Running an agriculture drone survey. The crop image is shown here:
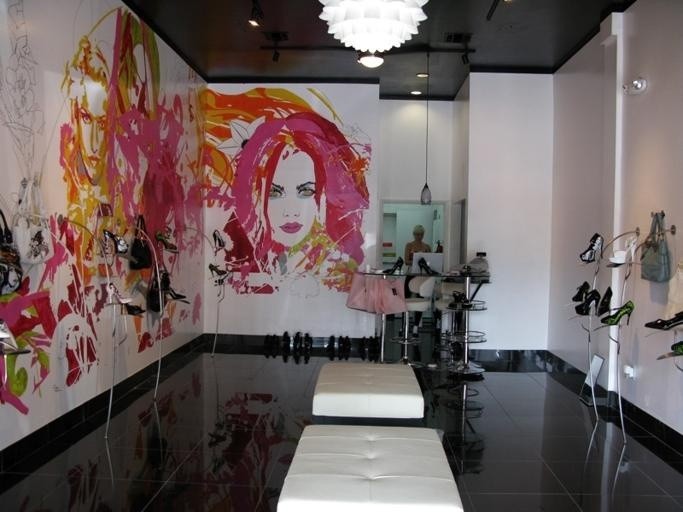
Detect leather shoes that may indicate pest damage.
[597,286,612,317]
[644,310,682,330]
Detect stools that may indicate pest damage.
[279,362,466,512]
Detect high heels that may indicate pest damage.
[575,289,602,316]
[155,231,178,250]
[600,300,635,326]
[108,282,133,304]
[120,301,146,316]
[571,281,590,302]
[418,257,442,276]
[212,229,225,247]
[383,255,404,274]
[580,232,605,262]
[103,229,128,254]
[208,262,226,277]
[164,288,187,300]
[608,237,638,264]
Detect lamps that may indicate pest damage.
[421,59,431,205]
[315,0,428,67]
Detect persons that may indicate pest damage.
[397,223,433,338]
[67,41,113,186]
[236,110,364,279]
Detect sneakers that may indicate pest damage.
[412,328,419,338]
[262,330,381,364]
[448,358,486,374]
[448,290,473,308]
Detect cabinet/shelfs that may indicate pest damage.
[442,278,493,375]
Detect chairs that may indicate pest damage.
[387,276,435,368]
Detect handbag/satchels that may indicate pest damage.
[442,258,491,277]
[12,180,55,265]
[0,209,24,297]
[146,261,171,313]
[129,215,153,270]
[345,272,409,315]
[640,213,672,283]
